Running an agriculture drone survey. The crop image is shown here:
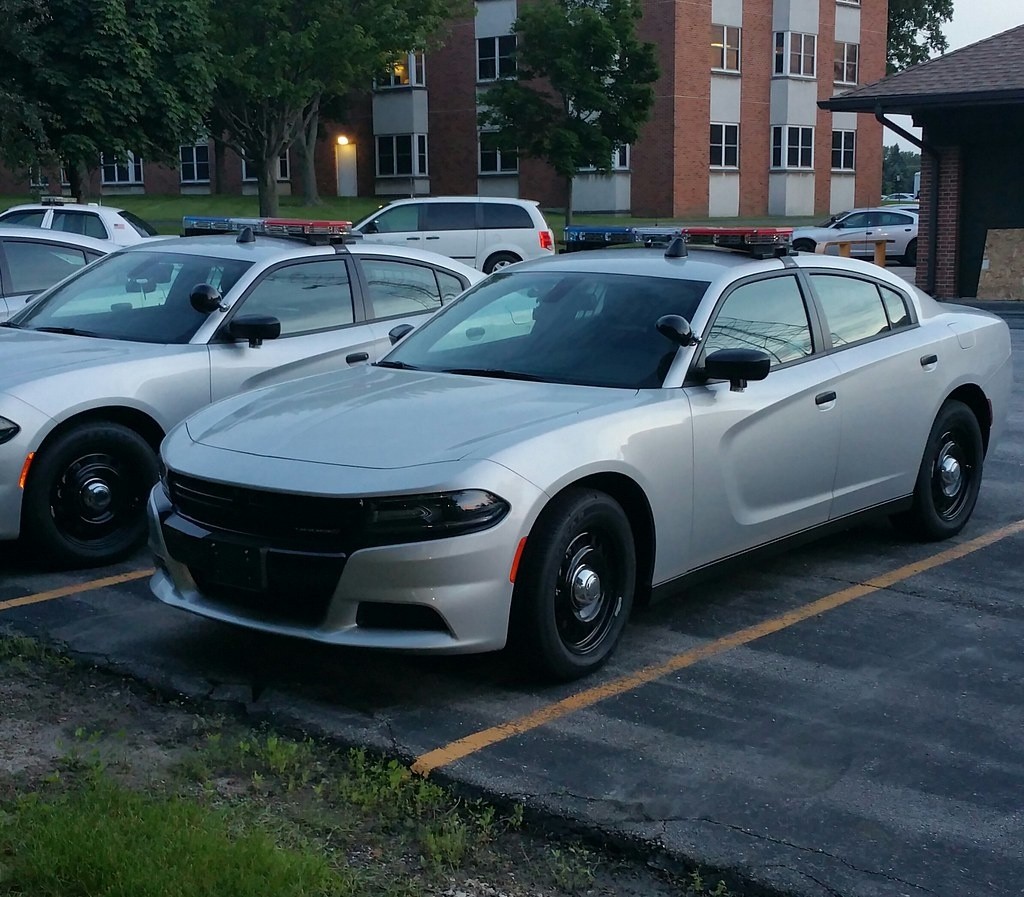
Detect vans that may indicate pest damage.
[356,194,557,275]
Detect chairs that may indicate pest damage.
[184,265,243,337]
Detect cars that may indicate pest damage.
[887,190,910,201]
[147,227,1010,679]
[4,198,155,246]
[2,227,109,314]
[794,205,919,270]
[2,225,495,566]
